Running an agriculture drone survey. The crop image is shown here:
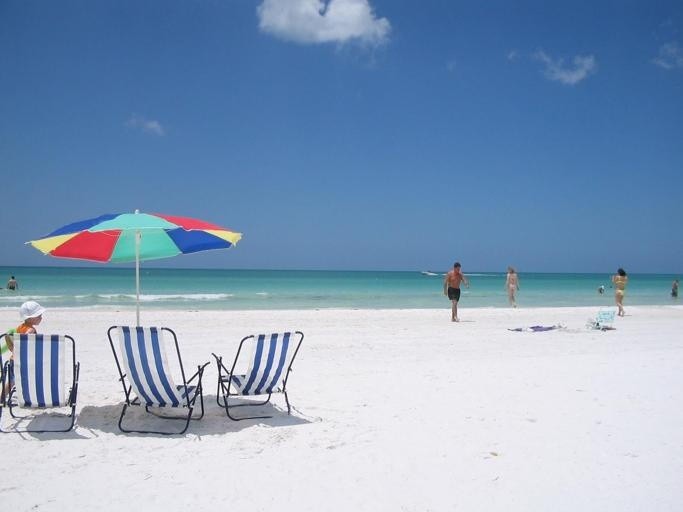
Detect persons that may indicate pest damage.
[6,275,19,291]
[671,279,679,296]
[504,267,520,308]
[443,262,470,322]
[611,267,628,317]
[599,285,605,297]
[0,301,45,400]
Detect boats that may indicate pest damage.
[421,271,433,275]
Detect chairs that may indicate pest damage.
[0,331,79,434]
[108,324,210,435]
[209,330,303,422]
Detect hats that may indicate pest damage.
[20,301,46,322]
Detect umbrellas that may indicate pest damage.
[25,208,243,326]
